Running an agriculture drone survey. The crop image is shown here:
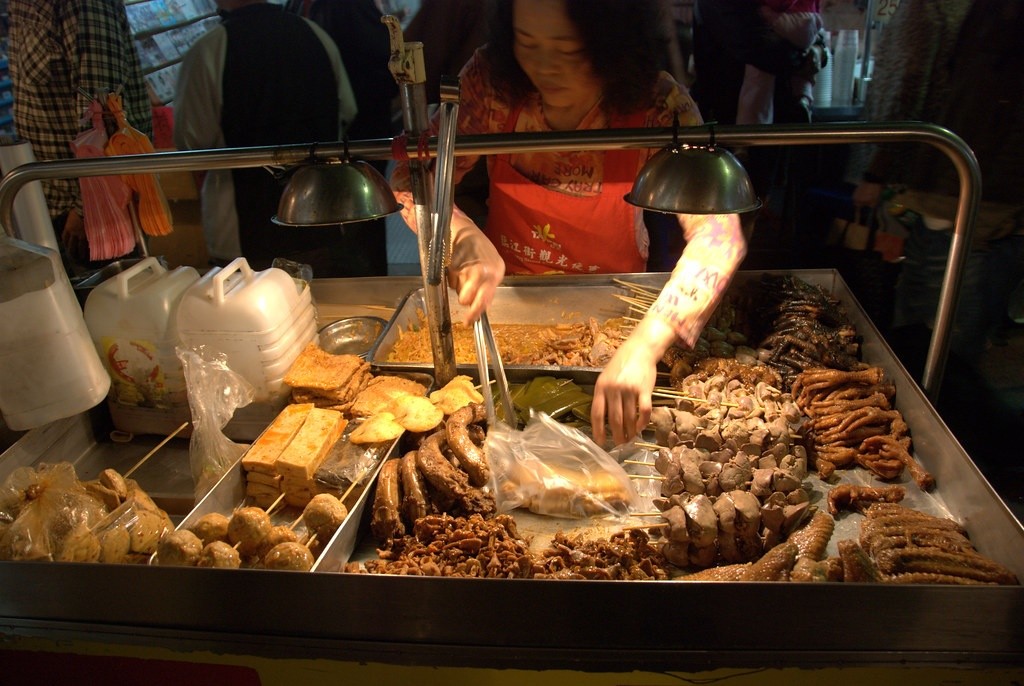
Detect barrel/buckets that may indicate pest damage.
[84,256,319,439]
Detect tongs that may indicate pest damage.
[474,307,518,435]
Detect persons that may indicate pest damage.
[7,0,156,263]
[173,0,358,279]
[387,0,750,449]
[283,0,406,200]
[686,0,830,161]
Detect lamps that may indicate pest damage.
[621,120,766,214]
[271,140,405,229]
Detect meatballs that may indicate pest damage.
[158,494,348,571]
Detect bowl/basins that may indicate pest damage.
[316,314,390,359]
